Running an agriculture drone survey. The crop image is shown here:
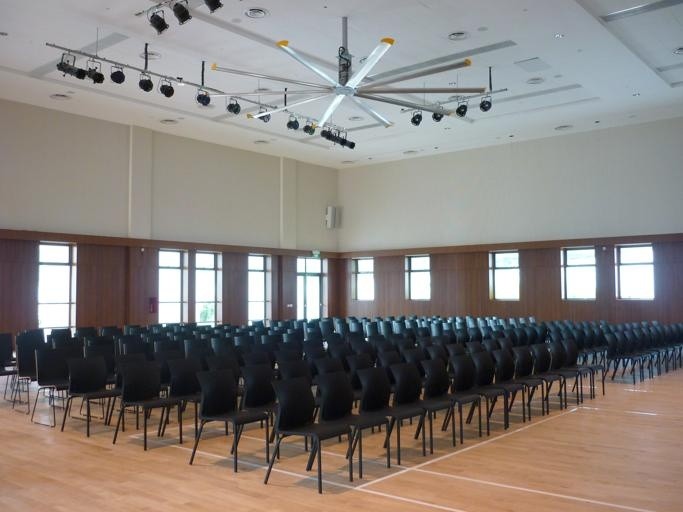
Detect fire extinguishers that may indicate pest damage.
[152,297,158,313]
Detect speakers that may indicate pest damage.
[327,204,338,231]
